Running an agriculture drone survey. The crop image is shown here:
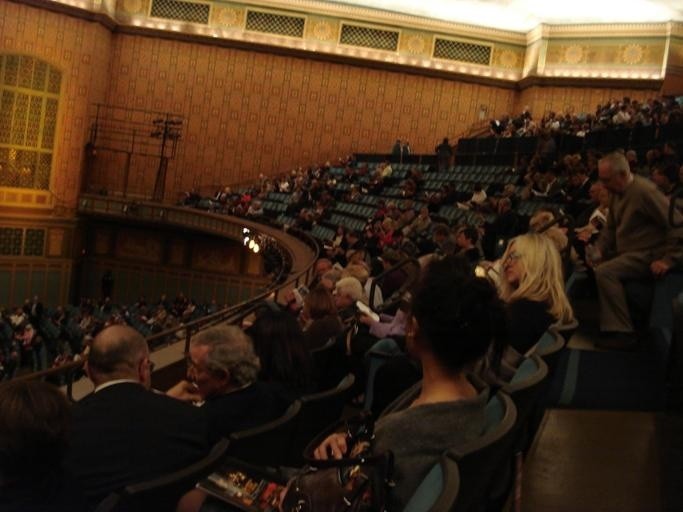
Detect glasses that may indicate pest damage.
[504,254,523,265]
[148,360,155,371]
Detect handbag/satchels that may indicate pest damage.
[281,410,381,512]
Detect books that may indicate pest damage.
[200,462,289,507]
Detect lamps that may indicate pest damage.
[238,227,269,256]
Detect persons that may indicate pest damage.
[298,287,344,352]
[340,263,368,304]
[403,141,412,157]
[332,277,367,327]
[167,326,291,442]
[0,295,41,379]
[41,292,97,372]
[320,271,341,287]
[593,141,682,340]
[41,328,208,512]
[393,139,401,157]
[0,380,70,464]
[315,257,332,276]
[487,91,683,154]
[97,287,195,328]
[322,228,491,261]
[492,234,577,382]
[529,213,577,275]
[435,136,453,159]
[357,290,414,340]
[180,261,530,512]
